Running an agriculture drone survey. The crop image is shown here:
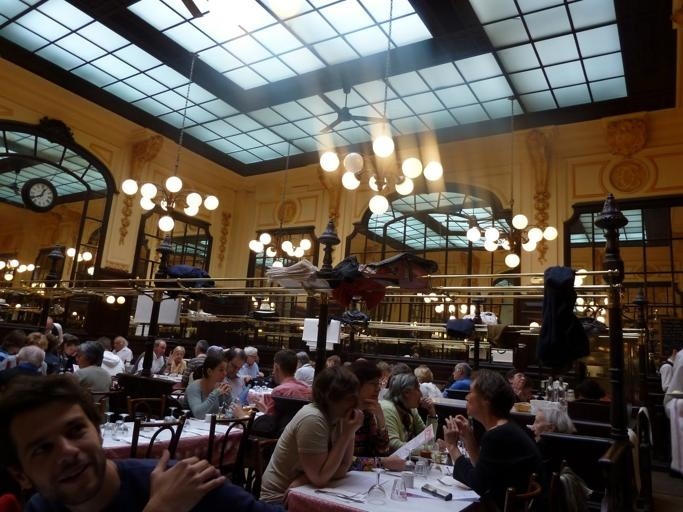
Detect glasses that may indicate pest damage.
[366,381,382,386]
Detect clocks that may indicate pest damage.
[21,177,58,213]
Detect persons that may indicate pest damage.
[231,349,313,465]
[394,363,412,374]
[188,345,224,387]
[378,362,392,388]
[348,360,406,471]
[132,339,177,376]
[258,365,365,512]
[659,343,683,391]
[223,348,248,408]
[0,328,80,375]
[414,365,442,398]
[506,369,533,402]
[0,373,286,512]
[325,355,367,367]
[378,373,438,455]
[294,351,315,386]
[180,340,209,388]
[97,336,126,377]
[442,363,473,397]
[376,365,384,389]
[575,378,605,401]
[72,341,112,410]
[663,349,683,419]
[443,369,541,512]
[531,406,577,443]
[238,346,266,378]
[112,336,133,365]
[183,357,233,418]
[171,346,187,373]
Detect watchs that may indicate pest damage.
[428,414,439,419]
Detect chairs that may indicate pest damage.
[551,459,567,507]
[130,415,186,459]
[207,410,256,483]
[94,398,107,423]
[251,438,279,494]
[504,472,541,512]
[126,394,166,422]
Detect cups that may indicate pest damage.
[182,410,190,432]
[103,412,114,437]
[405,447,415,466]
[120,414,129,435]
[415,461,426,474]
[391,479,407,501]
[368,468,386,503]
[169,407,177,423]
[114,421,124,439]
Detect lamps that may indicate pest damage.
[121,54,219,232]
[466,96,559,269]
[317,0,444,215]
[248,142,311,268]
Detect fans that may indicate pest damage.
[317,84,392,133]
